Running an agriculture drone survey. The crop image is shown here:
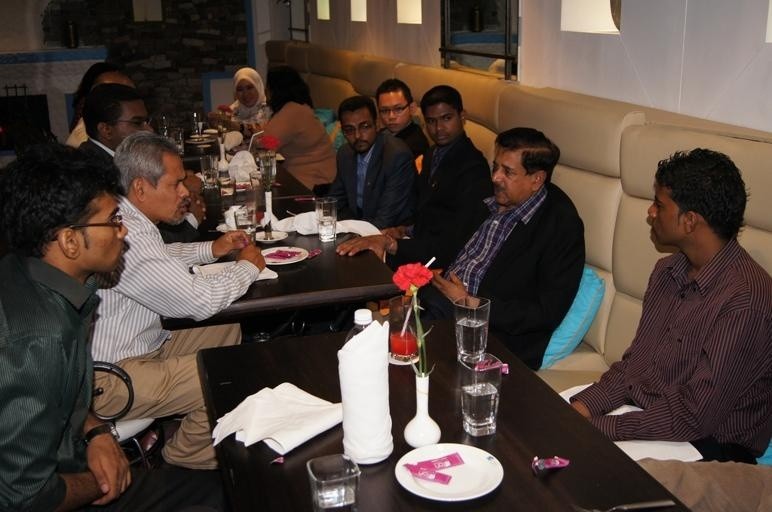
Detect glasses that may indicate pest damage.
[110,117,153,128]
[340,121,377,135]
[379,103,411,115]
[51,207,125,241]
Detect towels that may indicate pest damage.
[274,212,324,234]
[338,318,393,465]
[212,382,343,456]
[336,219,382,237]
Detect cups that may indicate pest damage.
[306,454,361,512]
[316,197,338,242]
[236,210,255,244]
[453,295,490,368]
[459,354,502,436]
[200,155,219,186]
[389,296,420,361]
[170,128,184,157]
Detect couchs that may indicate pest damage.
[263,41,771,393]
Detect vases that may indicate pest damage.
[403,373,441,449]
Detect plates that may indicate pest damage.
[186,138,215,144]
[261,247,309,265]
[395,443,503,501]
[260,150,276,177]
[256,231,287,243]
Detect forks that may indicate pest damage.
[573,499,675,511]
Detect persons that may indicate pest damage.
[569,148,772,465]
[253,65,337,196]
[327,95,417,230]
[0,141,183,512]
[310,78,430,197]
[90,130,265,505]
[226,67,274,143]
[77,82,155,197]
[66,62,137,149]
[332,126,586,372]
[633,458,772,512]
[381,85,494,277]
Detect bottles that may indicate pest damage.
[345,309,375,346]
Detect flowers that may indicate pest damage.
[389,261,439,378]
